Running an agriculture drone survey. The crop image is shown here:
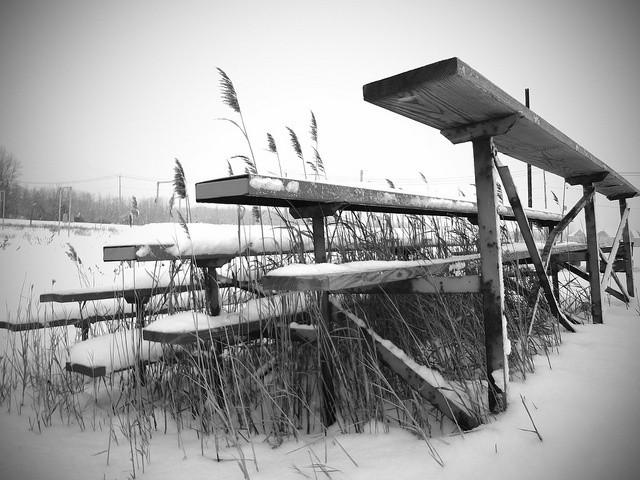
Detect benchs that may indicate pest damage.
[65,229,478,419]
[0,273,278,342]
[145,174,567,427]
[262,57,640,431]
